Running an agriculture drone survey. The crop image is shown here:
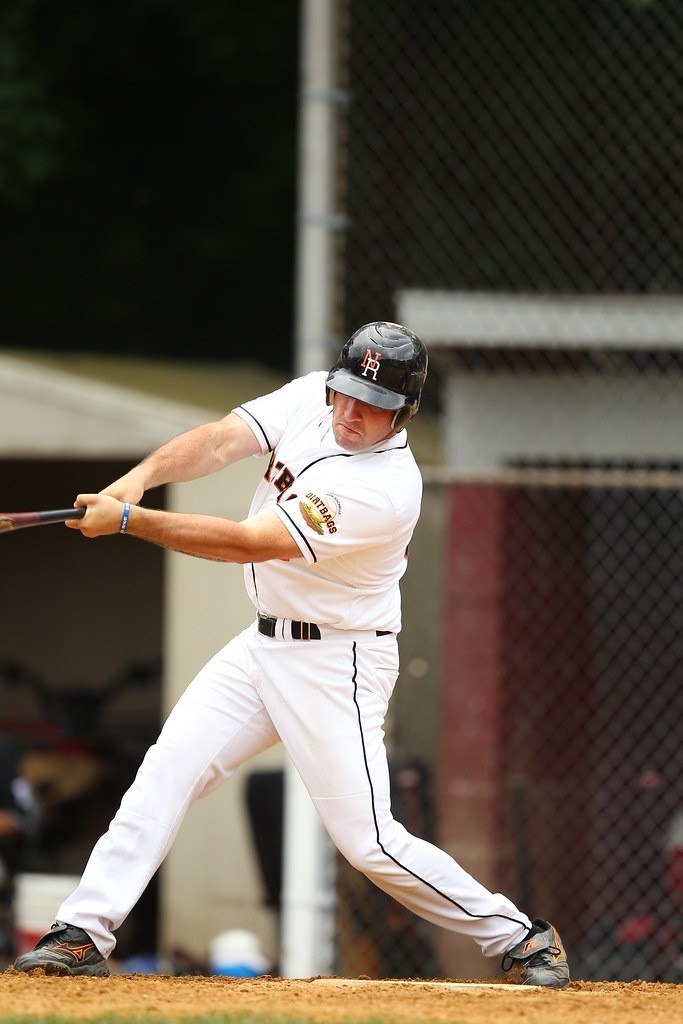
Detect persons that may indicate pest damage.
[14,321,570,989]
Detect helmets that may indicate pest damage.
[326,321,428,432]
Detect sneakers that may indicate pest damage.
[14,922,110,976]
[502,919,570,988]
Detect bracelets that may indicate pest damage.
[120,502,129,534]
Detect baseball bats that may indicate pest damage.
[0,506,86,535]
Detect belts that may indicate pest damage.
[258,615,392,640]
